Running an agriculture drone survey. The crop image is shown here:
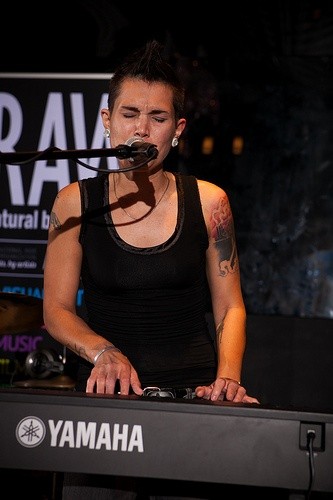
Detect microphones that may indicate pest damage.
[126,136,159,163]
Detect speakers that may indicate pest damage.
[205,312,333,412]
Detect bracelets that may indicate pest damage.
[92,345,121,364]
[218,376,241,384]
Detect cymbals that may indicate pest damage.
[18,375,78,389]
[0,291,45,334]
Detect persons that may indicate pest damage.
[37,38,261,410]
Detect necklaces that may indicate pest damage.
[113,168,170,222]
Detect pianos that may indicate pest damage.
[1,385,333,494]
